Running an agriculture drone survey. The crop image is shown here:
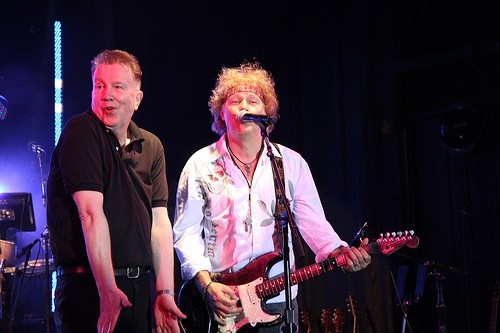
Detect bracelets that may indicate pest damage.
[156,290,175,296]
[203,280,212,300]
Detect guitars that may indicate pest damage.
[0,259,57,275]
[345,293,360,333]
[300,307,312,332]
[320,307,331,333]
[178,231,424,332]
[331,307,344,332]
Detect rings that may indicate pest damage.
[354,262,359,267]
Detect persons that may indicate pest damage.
[172,64,371,333]
[47,49,187,333]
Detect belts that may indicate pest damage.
[56,265,149,279]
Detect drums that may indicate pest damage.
[1,239,17,277]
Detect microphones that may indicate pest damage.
[27,141,46,153]
[350,222,369,248]
[16,239,38,259]
[238,110,274,124]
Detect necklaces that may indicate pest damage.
[227,143,260,231]
